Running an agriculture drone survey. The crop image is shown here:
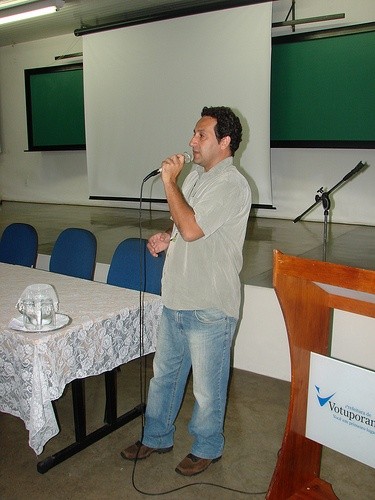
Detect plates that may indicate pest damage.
[7,313,70,333]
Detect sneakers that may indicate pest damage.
[120,440,173,461]
[175,453,222,477]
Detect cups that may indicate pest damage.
[16,283,58,329]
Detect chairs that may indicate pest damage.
[0,222,39,269]
[106,238,166,296]
[48,227,98,282]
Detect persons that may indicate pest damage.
[119,105,252,477]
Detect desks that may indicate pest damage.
[0,261,164,475]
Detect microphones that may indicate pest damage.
[143,152,195,182]
